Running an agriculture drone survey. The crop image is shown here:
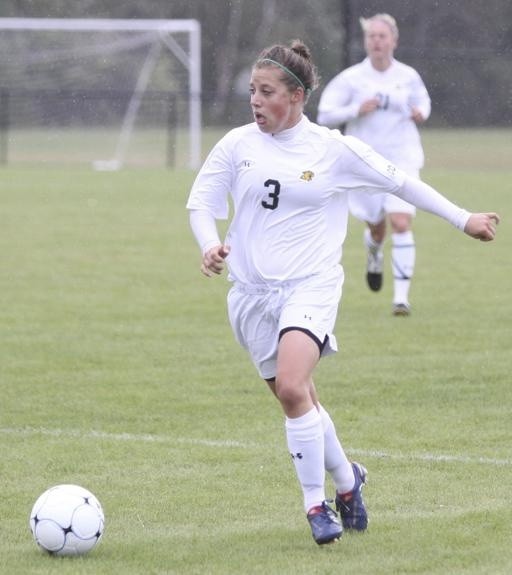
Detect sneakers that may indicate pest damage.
[367,270,383,293]
[307,503,345,547]
[333,460,370,532]
[390,300,411,317]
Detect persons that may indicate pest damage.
[184,38,502,550]
[312,10,435,318]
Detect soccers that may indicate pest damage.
[30,483,105,557]
